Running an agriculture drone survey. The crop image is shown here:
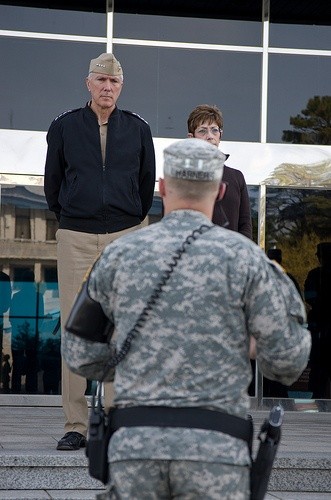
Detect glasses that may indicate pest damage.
[195,128,223,136]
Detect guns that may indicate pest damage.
[251,406,286,500]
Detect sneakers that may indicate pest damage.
[56,432,88,450]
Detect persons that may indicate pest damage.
[161,104,253,241]
[305,242,331,400]
[61,138,311,499]
[44,54,156,451]
[0,265,44,394]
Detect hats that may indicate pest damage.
[314,242,331,254]
[89,52,123,76]
[163,138,225,181]
